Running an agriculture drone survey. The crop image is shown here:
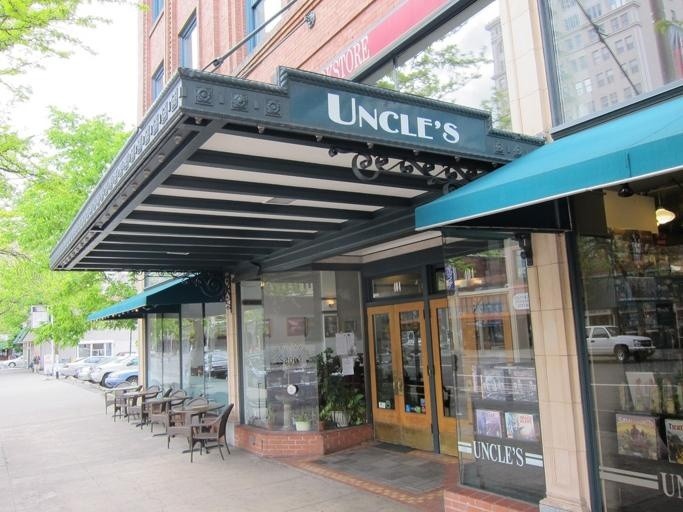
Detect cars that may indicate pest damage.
[7,355,25,368]
[585,324,656,363]
[204,349,228,375]
[61,352,139,387]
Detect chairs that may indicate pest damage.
[104,380,234,463]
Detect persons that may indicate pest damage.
[32,353,40,375]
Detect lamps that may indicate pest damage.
[656,193,676,227]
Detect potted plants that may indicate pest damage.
[294,374,366,431]
